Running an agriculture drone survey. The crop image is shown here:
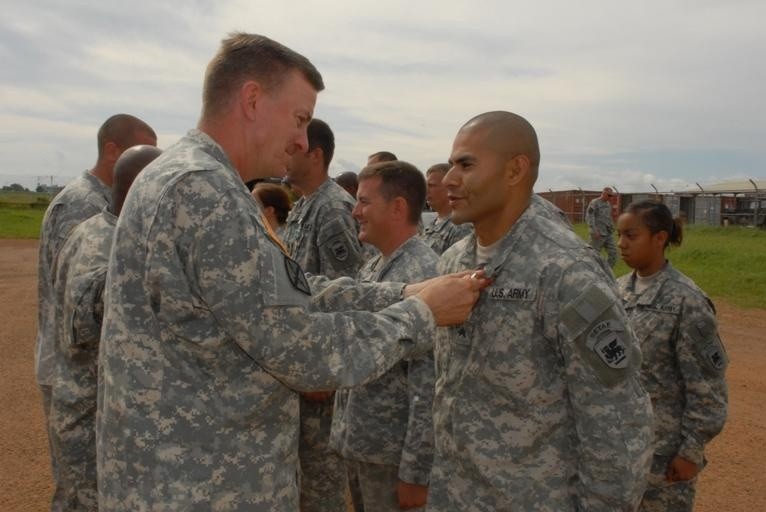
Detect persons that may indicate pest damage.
[48,145,167,511]
[34,115,156,511]
[604,198,729,511]
[96,29,491,511]
[239,118,578,510]
[582,185,618,271]
[430,109,656,512]
